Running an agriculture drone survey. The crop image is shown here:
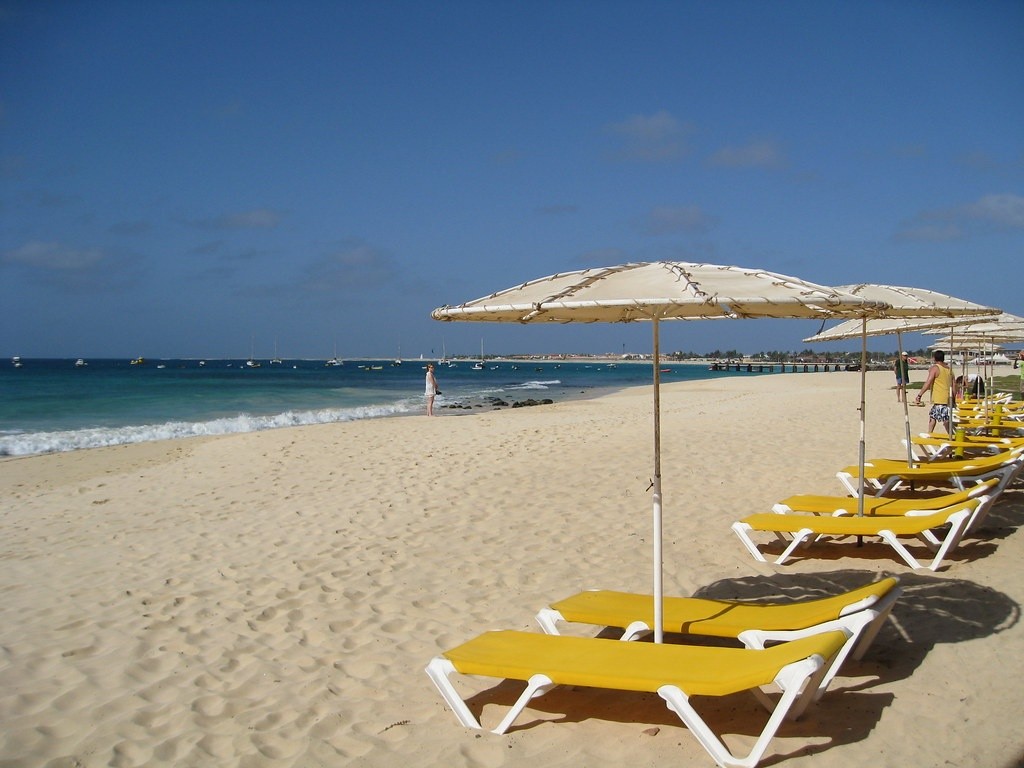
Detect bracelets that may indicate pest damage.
[918,395,921,397]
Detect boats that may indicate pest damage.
[12,356,23,368]
[76,358,88,367]
[131,357,145,365]
[325,344,343,367]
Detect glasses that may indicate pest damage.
[428,366,433,368]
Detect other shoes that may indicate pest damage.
[908,401,925,407]
[436,390,442,395]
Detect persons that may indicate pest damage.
[426,364,438,416]
[1014,351,1024,401]
[894,351,909,402]
[915,350,956,434]
[965,374,984,399]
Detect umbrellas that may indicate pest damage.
[428,261,894,644]
[828,284,1002,517]
[922,312,1024,436]
[802,316,999,491]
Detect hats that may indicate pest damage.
[1020,350,1024,356]
[901,351,908,356]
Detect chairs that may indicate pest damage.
[732,393,1024,571]
[425,626,854,768]
[536,575,903,702]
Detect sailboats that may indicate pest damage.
[472,337,484,369]
[270,335,281,364]
[389,337,402,368]
[439,337,450,366]
[247,335,260,368]
